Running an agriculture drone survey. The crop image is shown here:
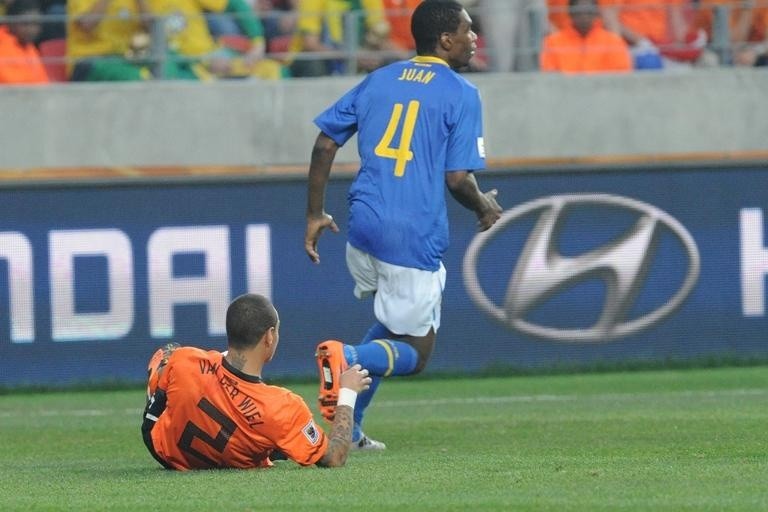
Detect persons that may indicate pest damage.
[142,293,373,472]
[3,1,768,83]
[306,0,503,450]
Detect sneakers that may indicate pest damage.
[316,340,349,424]
[348,434,388,452]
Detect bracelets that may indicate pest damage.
[336,387,358,410]
